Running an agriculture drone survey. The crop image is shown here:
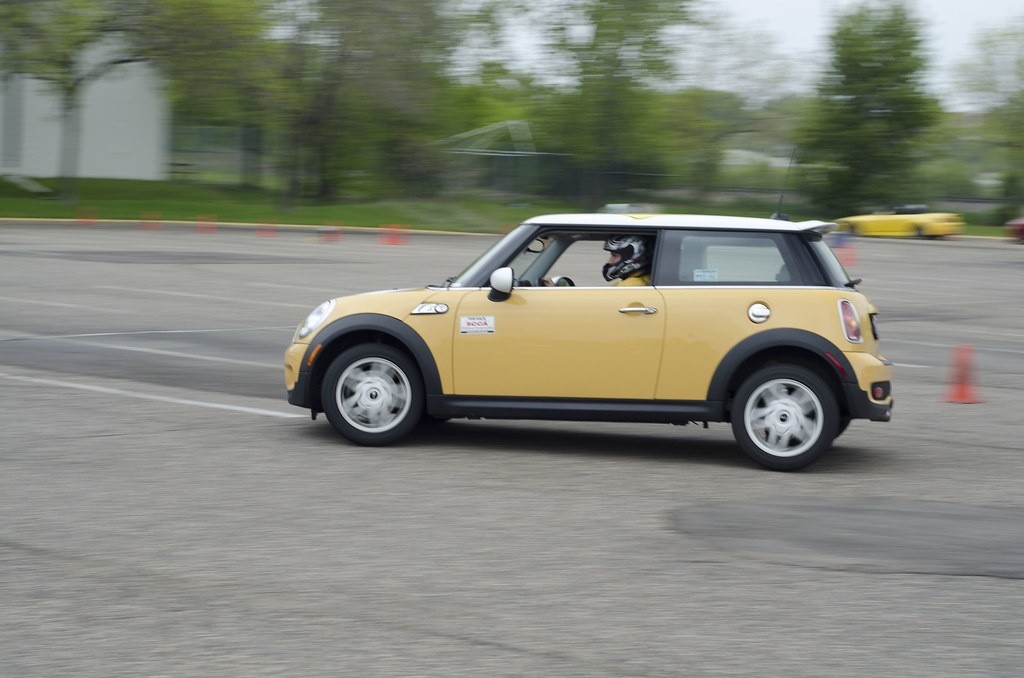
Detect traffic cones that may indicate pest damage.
[943,344,983,404]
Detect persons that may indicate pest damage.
[543,236,651,287]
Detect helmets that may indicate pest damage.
[602,234,651,282]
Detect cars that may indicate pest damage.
[283,211,895,472]
[829,203,969,239]
[1005,215,1024,240]
